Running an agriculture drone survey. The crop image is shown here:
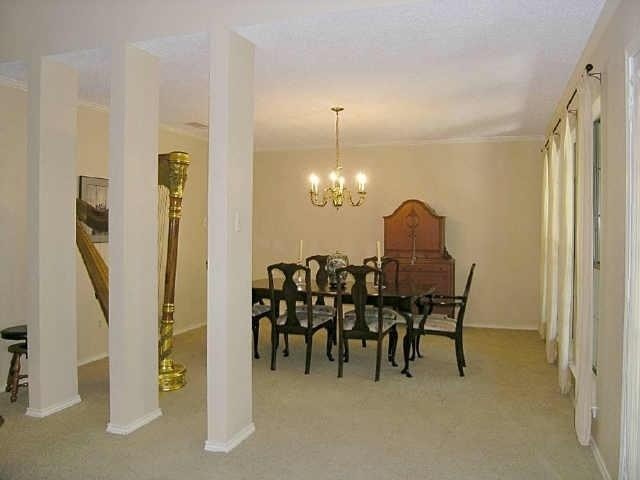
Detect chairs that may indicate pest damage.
[409,263,505,377]
[252,305,279,358]
[335,264,406,382]
[266,262,335,375]
[305,255,337,345]
[361,256,399,347]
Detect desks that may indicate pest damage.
[0,325,27,340]
[252,278,438,377]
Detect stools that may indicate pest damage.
[6,343,27,403]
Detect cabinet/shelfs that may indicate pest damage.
[382,258,455,319]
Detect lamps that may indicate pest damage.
[308,106,367,210]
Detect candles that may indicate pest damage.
[376,240,381,261]
[299,240,303,259]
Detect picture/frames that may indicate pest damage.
[79,176,109,244]
